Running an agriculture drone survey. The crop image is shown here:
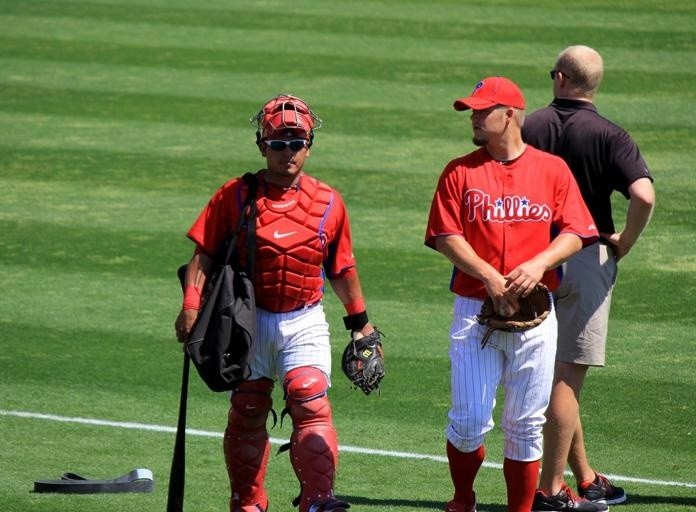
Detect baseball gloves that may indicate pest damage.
[341,327,384,396]
[477,281,551,349]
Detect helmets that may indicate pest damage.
[248,92,323,140]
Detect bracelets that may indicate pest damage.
[181,283,202,312]
[344,295,366,315]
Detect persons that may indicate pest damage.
[175,93,385,512]
[423,76,614,510]
[511,42,657,510]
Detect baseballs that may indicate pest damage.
[499,303,517,316]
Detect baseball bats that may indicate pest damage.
[167,345,189,511]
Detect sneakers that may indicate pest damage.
[532,482,609,512]
[577,471,626,504]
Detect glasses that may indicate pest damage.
[264,138,308,151]
[550,70,570,80]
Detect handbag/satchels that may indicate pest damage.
[178,255,257,392]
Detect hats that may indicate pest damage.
[454,76,526,112]
